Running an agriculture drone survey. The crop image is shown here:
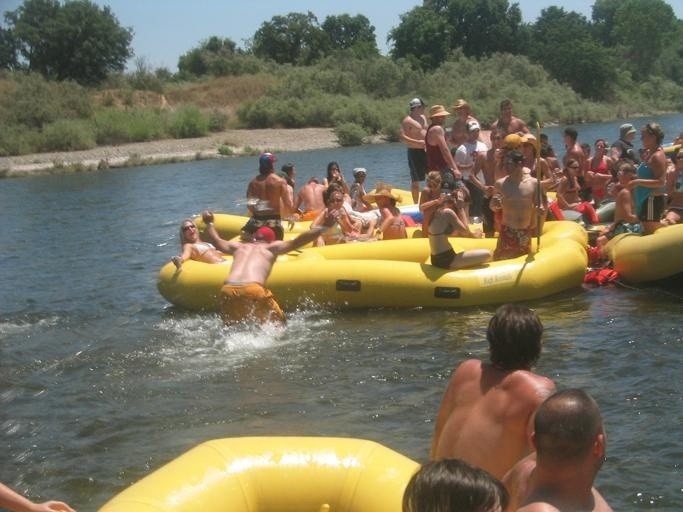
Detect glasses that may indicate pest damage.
[183,225,194,232]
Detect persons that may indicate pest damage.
[203,207,341,329]
[501,388,614,512]
[429,304,557,481]
[172,97,682,269]
[0,483,76,512]
[402,458,511,512]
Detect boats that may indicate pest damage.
[156,235,588,313]
[95,436,423,511]
[366,186,559,210]
[192,213,587,250]
[334,204,585,228]
[590,222,683,285]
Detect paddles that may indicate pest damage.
[535,121,541,249]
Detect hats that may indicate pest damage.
[562,127,577,139]
[500,134,536,151]
[259,152,277,162]
[255,226,275,240]
[362,182,402,203]
[409,97,481,132]
[619,123,636,138]
[352,167,367,175]
